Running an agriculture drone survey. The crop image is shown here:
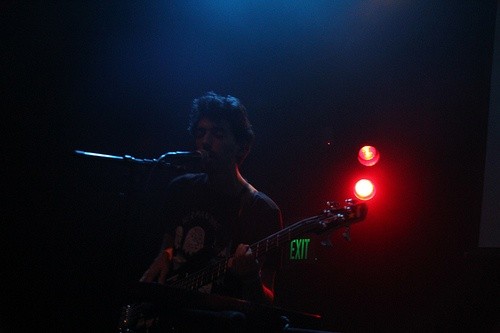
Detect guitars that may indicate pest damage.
[120,199,366,333]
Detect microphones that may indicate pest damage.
[160,149,210,163]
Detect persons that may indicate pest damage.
[126,93,284,332]
